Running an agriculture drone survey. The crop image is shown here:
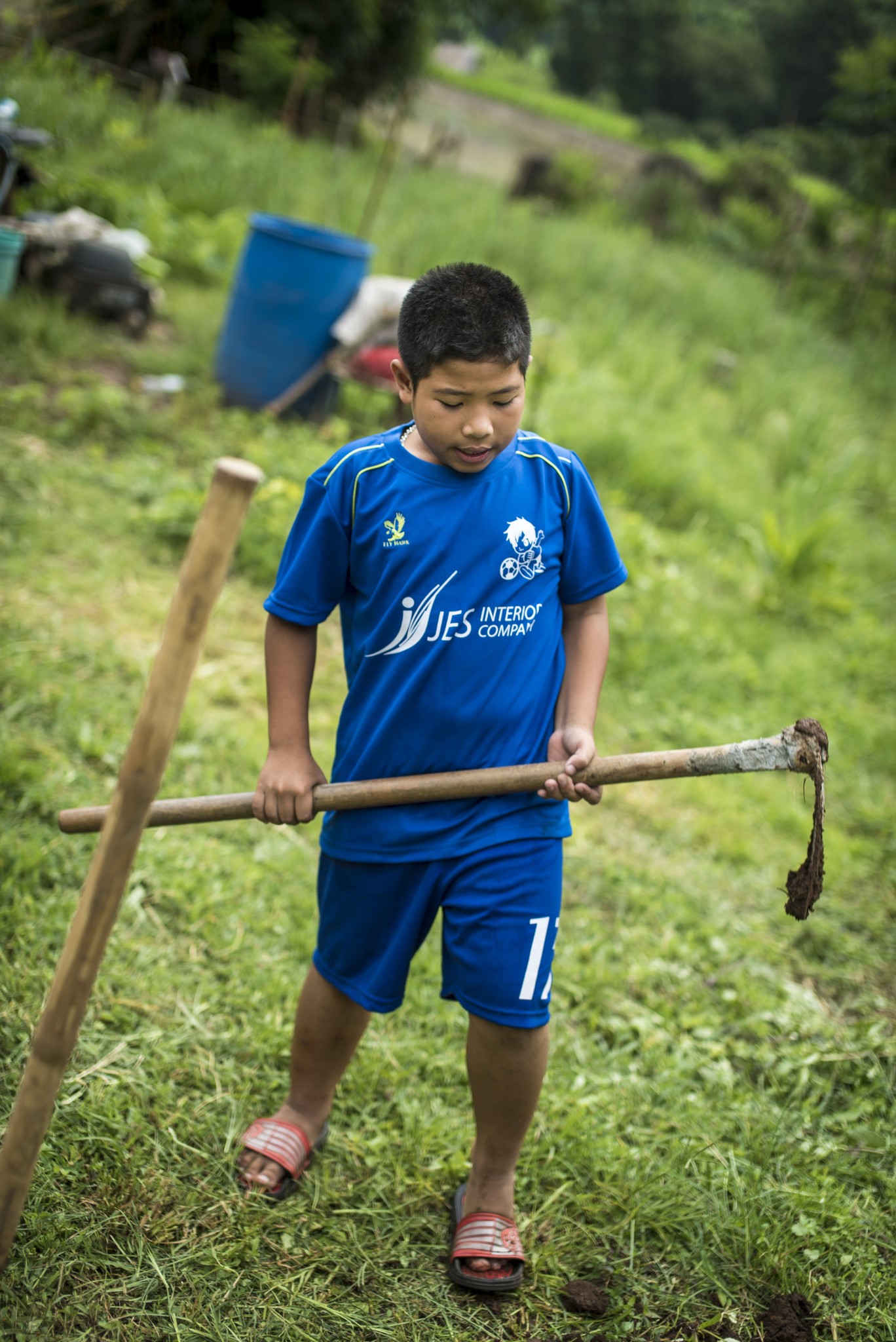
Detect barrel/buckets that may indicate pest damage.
[214,209,375,428]
[1,230,27,299]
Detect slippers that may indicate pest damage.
[235,1115,330,1199]
[449,1181,527,1293]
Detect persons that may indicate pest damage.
[236,261,630,1294]
